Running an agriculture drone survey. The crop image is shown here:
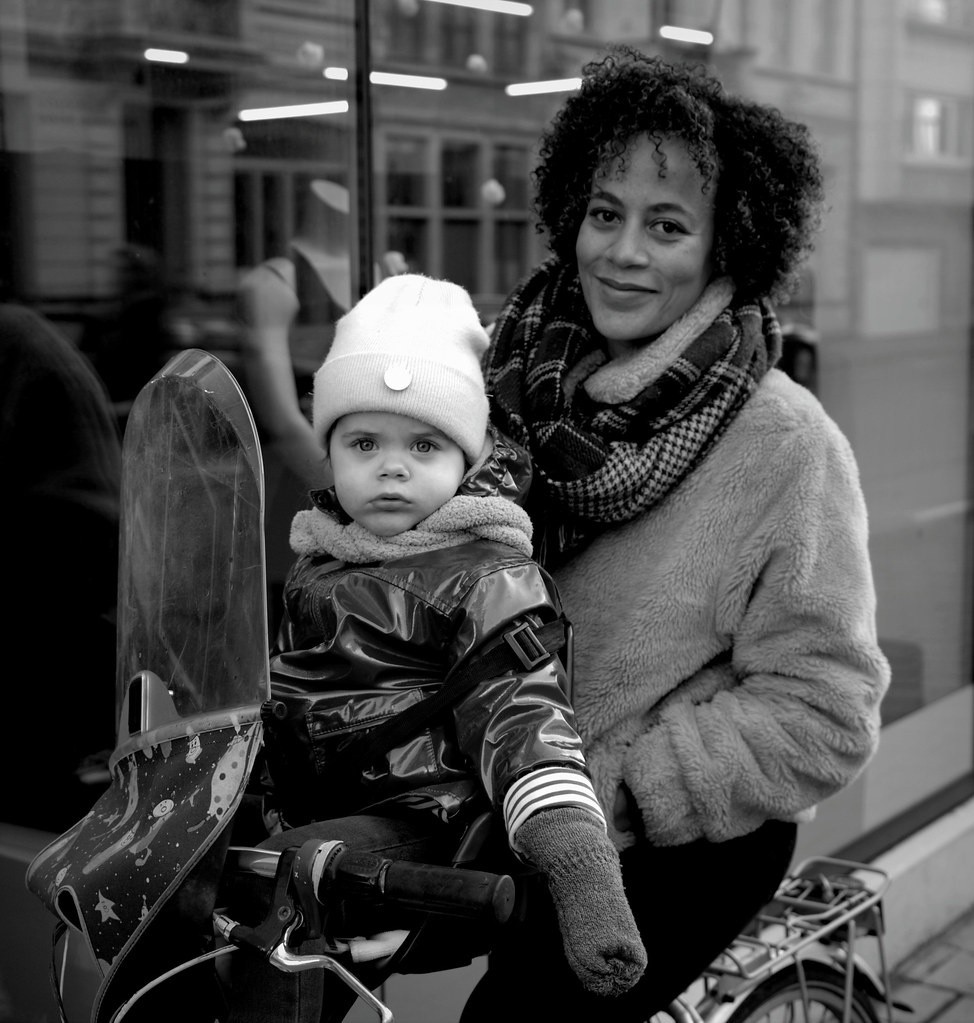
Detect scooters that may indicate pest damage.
[0,347,915,1023]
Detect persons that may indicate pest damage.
[185,45,891,1023]
[236,179,400,676]
[115,265,646,1023]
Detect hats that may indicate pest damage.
[312,273,491,465]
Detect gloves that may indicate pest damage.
[513,808,647,996]
